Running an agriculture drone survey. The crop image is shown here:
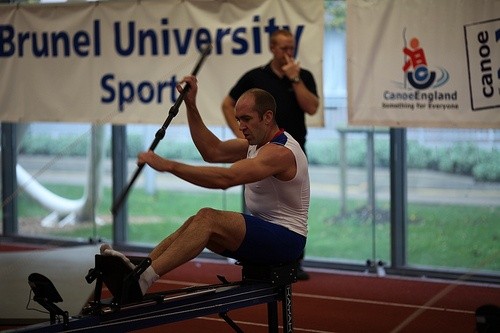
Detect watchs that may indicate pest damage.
[290,77,302,83]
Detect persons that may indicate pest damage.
[221,29,319,281]
[100,88,310,297]
